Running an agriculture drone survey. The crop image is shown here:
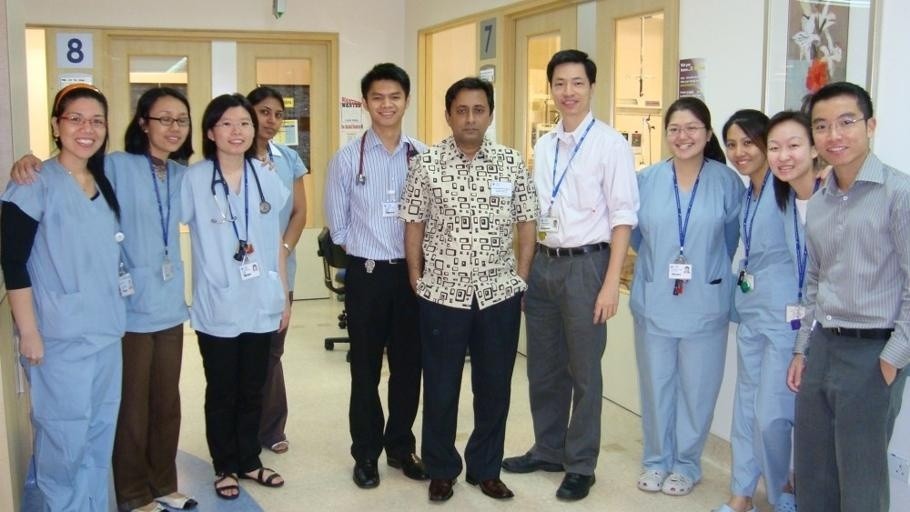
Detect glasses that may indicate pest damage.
[61,117,104,126]
[667,127,705,136]
[813,118,865,133]
[148,117,190,126]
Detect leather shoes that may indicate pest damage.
[353,450,595,501]
[130,496,198,512]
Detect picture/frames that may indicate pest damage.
[761,0,883,150]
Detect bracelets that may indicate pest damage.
[283,241,292,252]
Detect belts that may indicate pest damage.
[832,327,890,338]
[350,257,405,264]
[540,242,608,256]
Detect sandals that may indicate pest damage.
[238,468,284,486]
[215,472,240,499]
[269,439,288,453]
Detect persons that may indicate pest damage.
[400,77,540,503]
[253,264,257,271]
[715,105,801,512]
[552,220,557,227]
[626,93,747,497]
[177,91,293,499]
[328,62,435,492]
[0,82,132,512]
[683,266,689,274]
[780,80,910,512]
[765,107,833,315]
[500,49,643,505]
[245,85,313,458]
[10,87,199,512]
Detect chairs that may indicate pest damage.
[317,226,355,362]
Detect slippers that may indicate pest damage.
[712,503,757,512]
[775,492,797,512]
[638,470,693,495]
